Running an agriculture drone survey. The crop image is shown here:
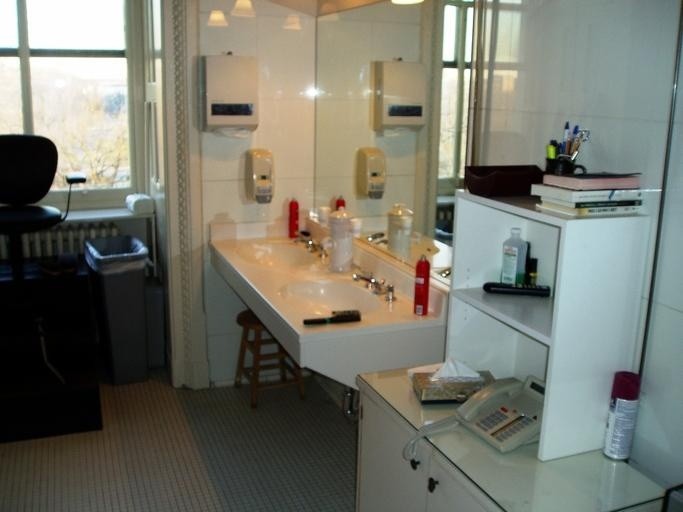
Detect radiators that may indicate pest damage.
[21,223,118,257]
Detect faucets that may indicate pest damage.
[352,273,387,295]
[440,268,452,278]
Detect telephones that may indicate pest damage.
[454,375,545,454]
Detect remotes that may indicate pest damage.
[483,283,550,297]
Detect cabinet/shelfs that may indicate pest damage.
[443,190,652,461]
[354,393,498,512]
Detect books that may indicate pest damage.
[529,170,642,215]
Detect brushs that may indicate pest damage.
[301,310,361,325]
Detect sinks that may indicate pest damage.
[278,280,382,314]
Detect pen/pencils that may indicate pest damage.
[546,118,589,162]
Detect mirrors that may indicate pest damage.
[316,0,477,284]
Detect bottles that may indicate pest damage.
[500,227,539,288]
[327,206,354,275]
[601,371,640,459]
[413,255,431,316]
[287,199,299,239]
[335,196,345,209]
[386,203,413,262]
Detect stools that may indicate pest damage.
[235,309,308,408]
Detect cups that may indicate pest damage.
[544,158,588,177]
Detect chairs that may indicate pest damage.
[1,135,61,277]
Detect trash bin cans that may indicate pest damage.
[84,234,149,388]
